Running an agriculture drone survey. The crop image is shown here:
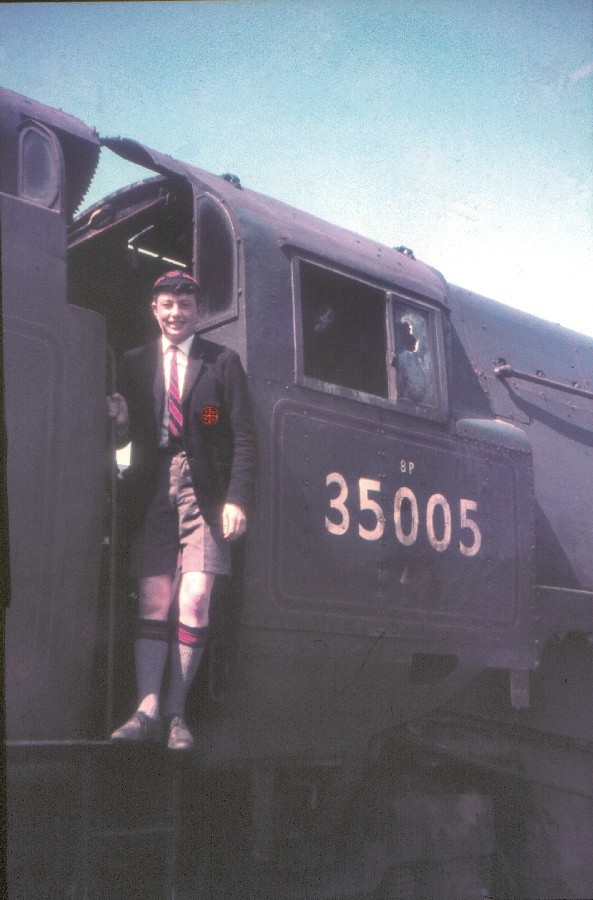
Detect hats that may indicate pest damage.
[153,270,201,293]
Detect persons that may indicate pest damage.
[105,270,259,752]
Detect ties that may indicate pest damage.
[168,346,183,441]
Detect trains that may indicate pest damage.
[0,84,593,900]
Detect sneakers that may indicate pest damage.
[165,712,195,751]
[111,711,163,745]
